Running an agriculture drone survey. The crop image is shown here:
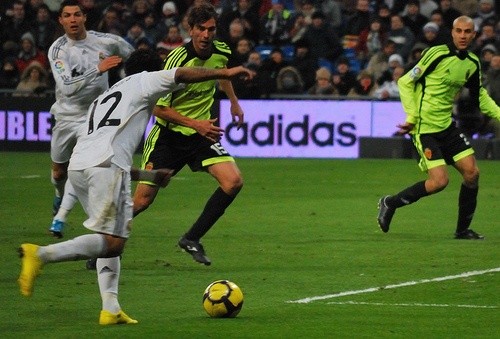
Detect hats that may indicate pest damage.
[423,22,439,34]
[388,54,403,67]
[316,66,330,80]
[162,2,176,14]
[311,12,327,20]
[20,32,35,47]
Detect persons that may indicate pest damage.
[376,15,500,240]
[47,0,135,238]
[85,5,244,266]
[15,48,256,325]
[0,0,500,106]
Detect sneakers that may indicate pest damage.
[453,229,484,241]
[377,195,395,233]
[175,237,212,267]
[98,309,139,326]
[47,219,65,240]
[85,258,96,271]
[51,196,62,215]
[15,241,46,299]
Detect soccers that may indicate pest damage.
[203,279,243,318]
